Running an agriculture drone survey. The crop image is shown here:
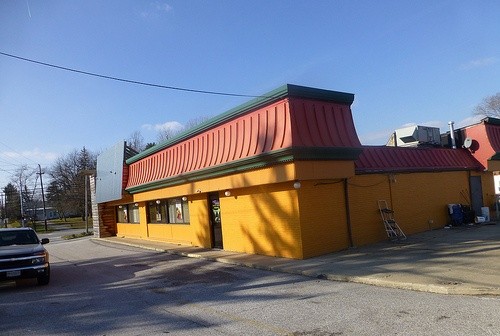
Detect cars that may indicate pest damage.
[0,227,51,285]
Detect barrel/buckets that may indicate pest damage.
[481,207,490,222]
[474,216,486,223]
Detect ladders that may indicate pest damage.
[378,200,407,241]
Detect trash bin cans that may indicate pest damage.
[460,204,472,224]
[448,203,464,226]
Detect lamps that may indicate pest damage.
[293,181,301,189]
[182,196,187,201]
[225,190,230,196]
